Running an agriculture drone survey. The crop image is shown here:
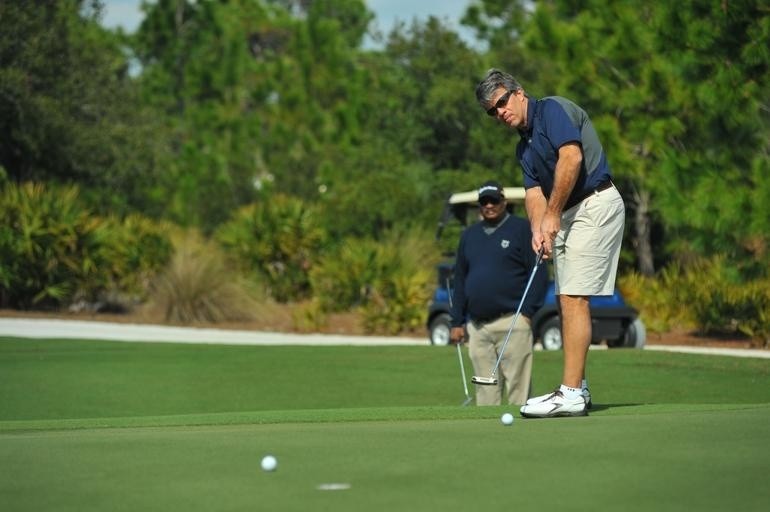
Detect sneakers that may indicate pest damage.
[520,387,591,418]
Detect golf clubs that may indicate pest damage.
[445,278,472,406]
[471,245,544,385]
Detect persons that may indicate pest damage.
[449,180,549,408]
[476,67,628,419]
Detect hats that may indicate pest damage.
[479,182,505,202]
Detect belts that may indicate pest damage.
[557,180,613,212]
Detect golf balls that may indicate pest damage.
[501,413,512,425]
[261,456,276,471]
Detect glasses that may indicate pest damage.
[486,92,513,119]
